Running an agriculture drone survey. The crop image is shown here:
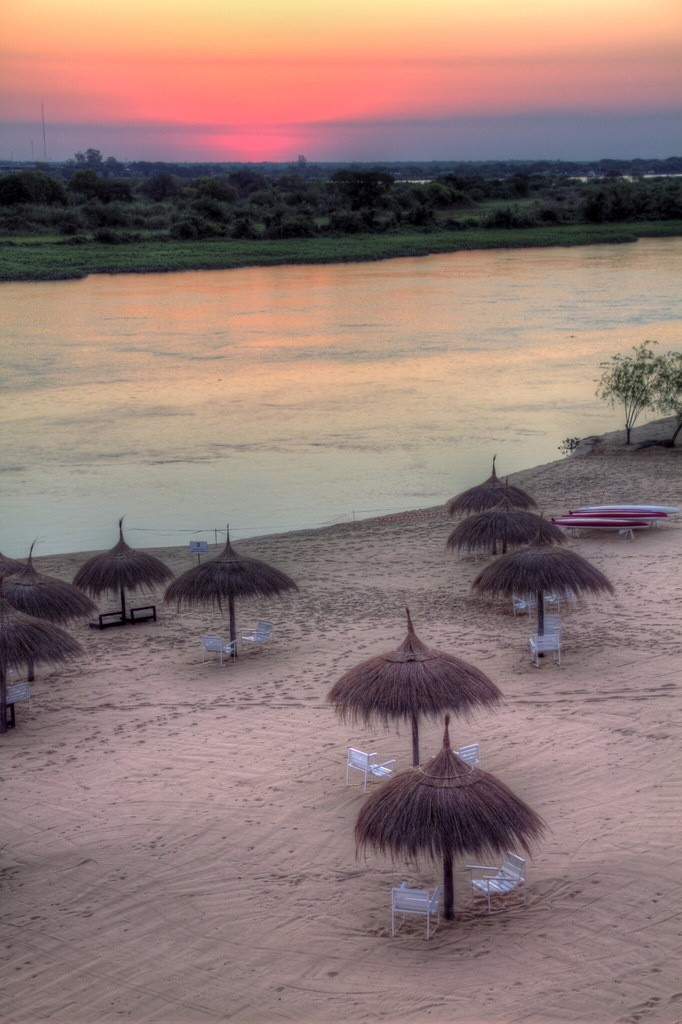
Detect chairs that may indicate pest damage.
[391,881,440,940]
[543,589,561,614]
[89,611,124,630]
[529,634,561,667]
[240,618,275,654]
[347,747,396,793]
[512,589,531,619]
[202,636,237,667]
[544,613,562,636]
[432,743,480,768]
[120,605,157,625]
[5,683,31,722]
[465,851,528,915]
[460,541,485,561]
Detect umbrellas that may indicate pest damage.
[71,517,175,626]
[448,454,539,517]
[444,476,568,554]
[325,606,504,767]
[353,714,551,921]
[164,522,299,658]
[471,511,614,658]
[0,542,101,682]
[0,592,84,732]
[0,551,26,580]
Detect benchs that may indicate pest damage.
[547,504,681,541]
